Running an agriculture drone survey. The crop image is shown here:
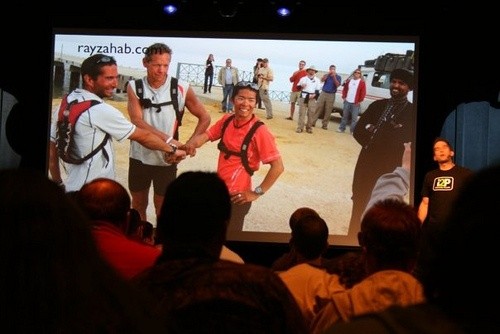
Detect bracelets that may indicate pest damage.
[166,137,173,144]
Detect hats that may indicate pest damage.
[305,65,318,74]
[389,68,414,91]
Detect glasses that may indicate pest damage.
[235,81,260,91]
[96,56,117,65]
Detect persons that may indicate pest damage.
[417,137,472,231]
[0,160,500,334]
[46,43,414,236]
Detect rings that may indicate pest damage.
[238,194,241,198]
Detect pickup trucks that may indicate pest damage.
[330,65,417,121]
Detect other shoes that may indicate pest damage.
[218,110,226,113]
[336,129,345,133]
[295,128,302,133]
[306,128,312,133]
[322,127,328,130]
[228,110,232,114]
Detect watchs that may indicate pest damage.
[169,143,178,154]
[254,186,264,195]
